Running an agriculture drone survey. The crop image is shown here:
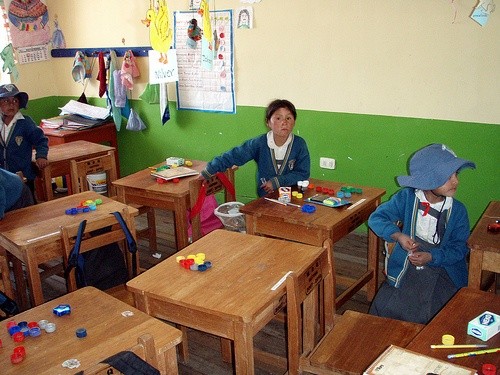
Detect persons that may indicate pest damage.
[0,83,49,189]
[0,168,34,221]
[368,144,477,326]
[194,99,311,197]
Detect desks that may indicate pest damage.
[126,229,322,375]
[238,178,386,337]
[404,285,500,375]
[0,286,183,375]
[32,139,116,202]
[466,200,500,293]
[111,160,239,251]
[38,123,121,197]
[0,191,140,311]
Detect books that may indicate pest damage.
[363,344,479,375]
[151,166,199,181]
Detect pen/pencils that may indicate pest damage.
[447,348,500,359]
[430,344,487,348]
[146,167,156,170]
[264,197,286,205]
[287,202,301,208]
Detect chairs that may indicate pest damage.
[60,207,189,365]
[188,165,236,243]
[286,238,426,375]
[70,150,157,254]
[82,332,161,375]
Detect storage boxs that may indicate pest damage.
[467,311,500,341]
[279,186,291,202]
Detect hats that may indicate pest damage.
[0,84,28,109]
[397,143,476,190]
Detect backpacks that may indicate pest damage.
[187,173,235,244]
[65,212,137,291]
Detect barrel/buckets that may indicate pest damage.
[86,172,108,193]
